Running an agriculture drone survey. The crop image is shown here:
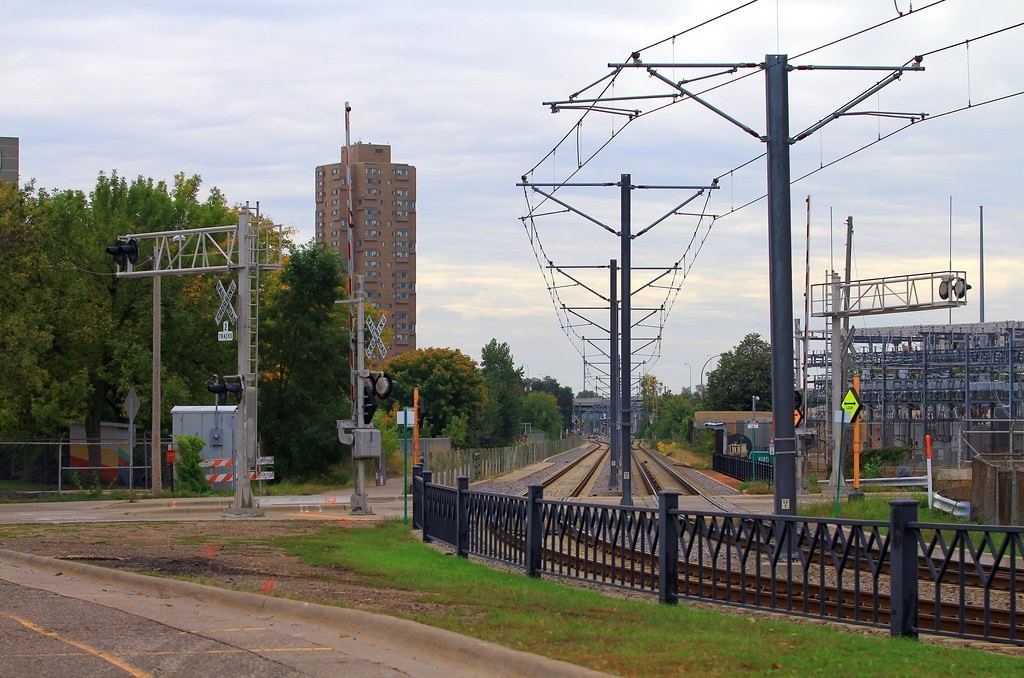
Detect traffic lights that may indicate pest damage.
[105,240,126,266]
[120,239,138,264]
[207,377,227,400]
[225,377,242,403]
